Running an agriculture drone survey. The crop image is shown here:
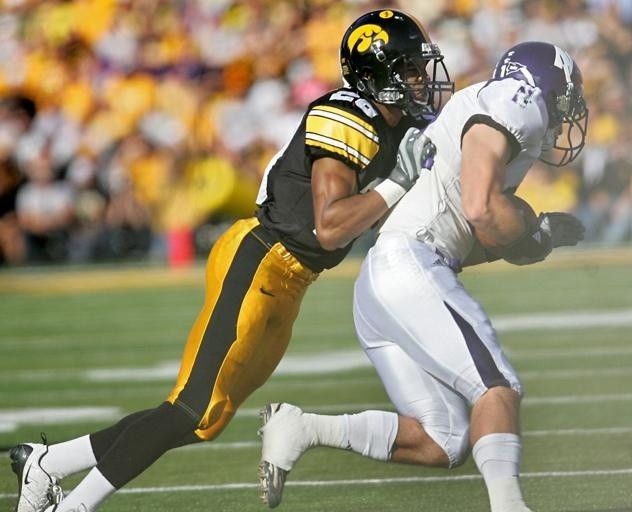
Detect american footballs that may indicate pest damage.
[472,191,542,261]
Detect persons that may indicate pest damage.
[10,7,456,512]
[1,1,632,275]
[256,38,592,512]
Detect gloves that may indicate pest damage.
[498,227,552,266]
[386,126,438,191]
[538,210,585,250]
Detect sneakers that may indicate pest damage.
[257,401,303,509]
[9,432,62,512]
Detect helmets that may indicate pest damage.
[339,9,456,130]
[492,42,589,169]
